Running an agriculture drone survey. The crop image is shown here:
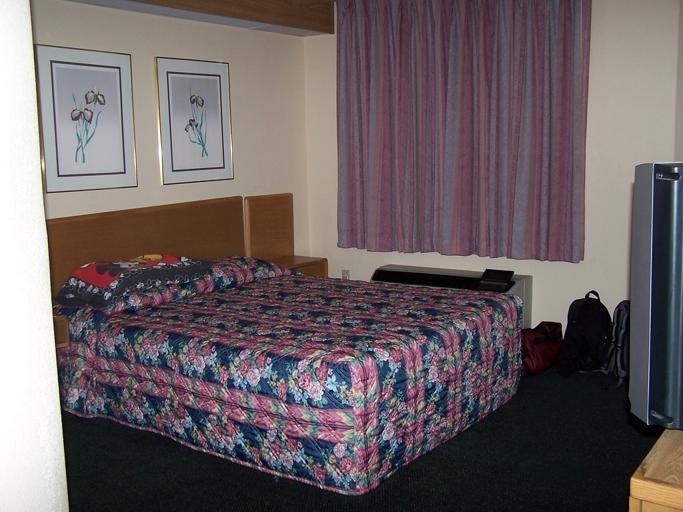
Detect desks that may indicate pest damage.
[628,429,683,511]
[370,264,532,333]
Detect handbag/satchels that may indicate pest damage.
[516,320,565,375]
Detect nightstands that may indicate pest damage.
[52,311,70,349]
[264,256,328,280]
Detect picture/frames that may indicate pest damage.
[34,44,138,193]
[153,56,234,185]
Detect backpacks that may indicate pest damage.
[609,299,632,379]
[560,288,614,378]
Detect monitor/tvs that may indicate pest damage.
[629,163,683,431]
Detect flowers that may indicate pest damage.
[69,89,106,165]
[184,87,210,158]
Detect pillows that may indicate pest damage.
[202,254,305,294]
[59,253,213,310]
[52,278,213,322]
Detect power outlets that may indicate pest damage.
[341,269,350,281]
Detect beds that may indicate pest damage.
[46,192,523,496]
